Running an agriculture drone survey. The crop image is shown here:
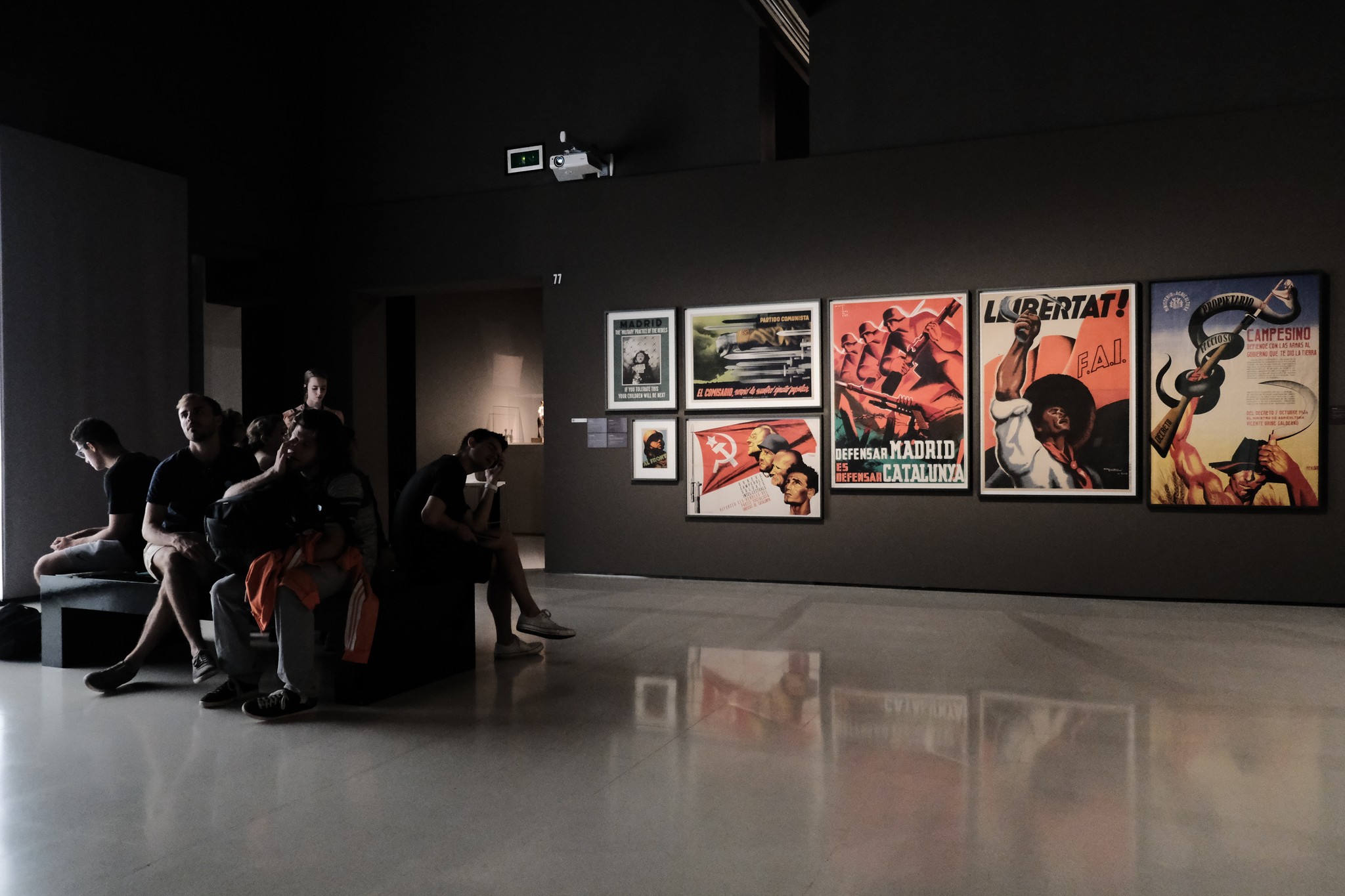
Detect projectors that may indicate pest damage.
[550,152,611,183]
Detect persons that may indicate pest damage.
[201,409,384,721]
[393,429,577,660]
[220,368,344,474]
[32,418,162,586]
[86,392,262,692]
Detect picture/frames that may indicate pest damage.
[828,289,971,492]
[631,417,677,481]
[975,280,1143,503]
[601,306,678,410]
[685,415,824,520]
[1138,268,1327,510]
[682,299,822,410]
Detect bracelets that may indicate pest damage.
[484,483,498,494]
[455,523,460,531]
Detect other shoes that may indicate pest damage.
[493,634,544,658]
[241,688,317,722]
[83,660,141,693]
[516,608,576,639]
[199,680,260,707]
[192,649,220,684]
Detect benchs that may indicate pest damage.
[40,572,475,710]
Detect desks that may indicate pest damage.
[501,443,545,534]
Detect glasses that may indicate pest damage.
[75,441,95,459]
